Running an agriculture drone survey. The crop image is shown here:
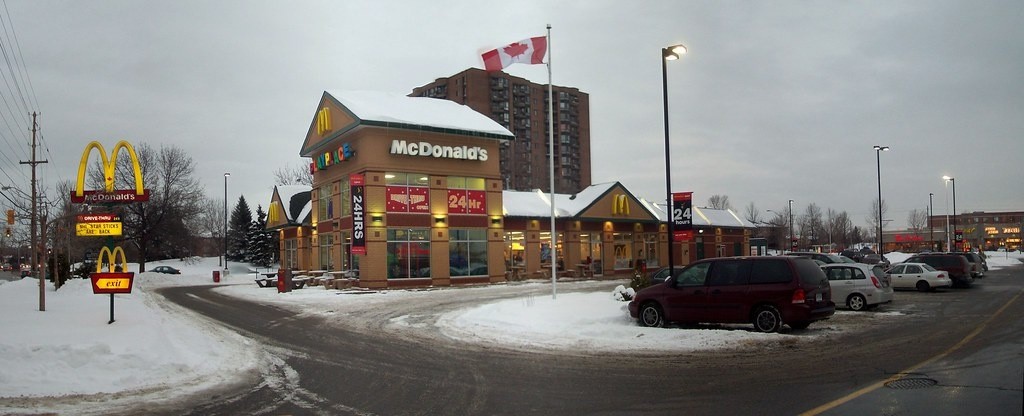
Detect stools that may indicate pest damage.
[520,273,528,280]
[567,270,575,278]
[255,271,357,290]
[586,270,596,278]
[505,271,511,281]
[533,272,542,279]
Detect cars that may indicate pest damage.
[147,265,182,276]
[637,250,952,311]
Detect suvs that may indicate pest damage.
[628,255,835,333]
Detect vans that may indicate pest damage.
[901,252,983,288]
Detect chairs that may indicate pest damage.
[830,271,836,280]
[838,271,846,279]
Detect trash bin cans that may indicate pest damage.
[277,269,293,293]
[213,270,220,283]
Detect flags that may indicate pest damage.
[481,36,547,73]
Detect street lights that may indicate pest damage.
[929,192,934,250]
[223,172,230,275]
[943,175,956,251]
[788,199,793,251]
[873,145,890,261]
[2,185,38,278]
[660,44,687,277]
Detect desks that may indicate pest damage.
[542,264,561,276]
[509,266,523,280]
[575,263,587,276]
[261,271,348,286]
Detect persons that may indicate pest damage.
[558,257,564,271]
[977,249,986,258]
[508,252,524,281]
